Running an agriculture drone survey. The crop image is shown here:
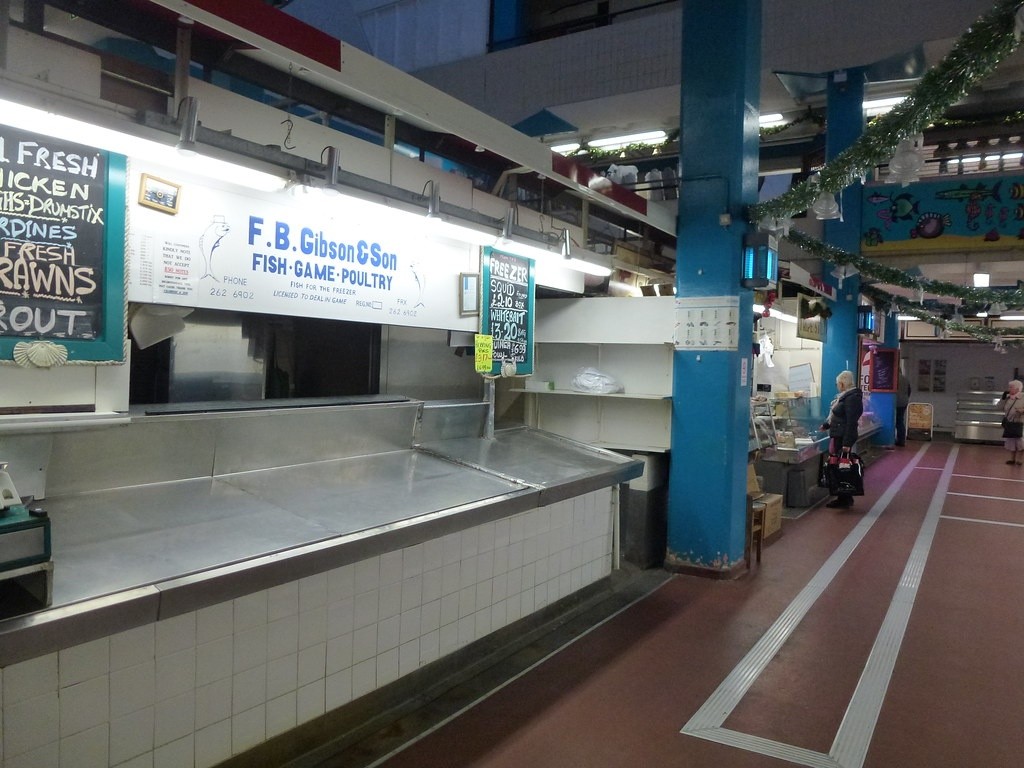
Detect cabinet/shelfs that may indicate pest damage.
[507,341,674,453]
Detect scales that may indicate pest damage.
[0,461,22,514]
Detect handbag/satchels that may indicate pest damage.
[1001,417,1007,428]
[830,452,864,496]
[817,450,864,487]
[1002,413,1023,438]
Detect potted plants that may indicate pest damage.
[556,144,681,163]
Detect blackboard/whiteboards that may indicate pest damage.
[479,246,535,376]
[0,123,128,368]
[868,348,897,393]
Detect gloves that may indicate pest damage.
[1002,391,1009,399]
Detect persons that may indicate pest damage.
[894,365,912,448]
[819,370,864,507]
[995,380,1024,465]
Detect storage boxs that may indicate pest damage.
[756,494,783,540]
[774,391,794,399]
[524,381,554,390]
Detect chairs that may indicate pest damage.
[745,492,766,569]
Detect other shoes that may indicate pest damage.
[895,441,905,446]
[826,502,850,509]
[1006,460,1015,464]
[831,500,854,506]
[1017,460,1022,465]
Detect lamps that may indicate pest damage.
[422,180,444,226]
[171,94,202,158]
[560,226,572,260]
[500,206,517,245]
[856,305,876,333]
[741,230,782,293]
[320,146,342,200]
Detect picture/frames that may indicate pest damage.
[137,172,184,215]
[458,267,482,317]
[611,238,675,273]
